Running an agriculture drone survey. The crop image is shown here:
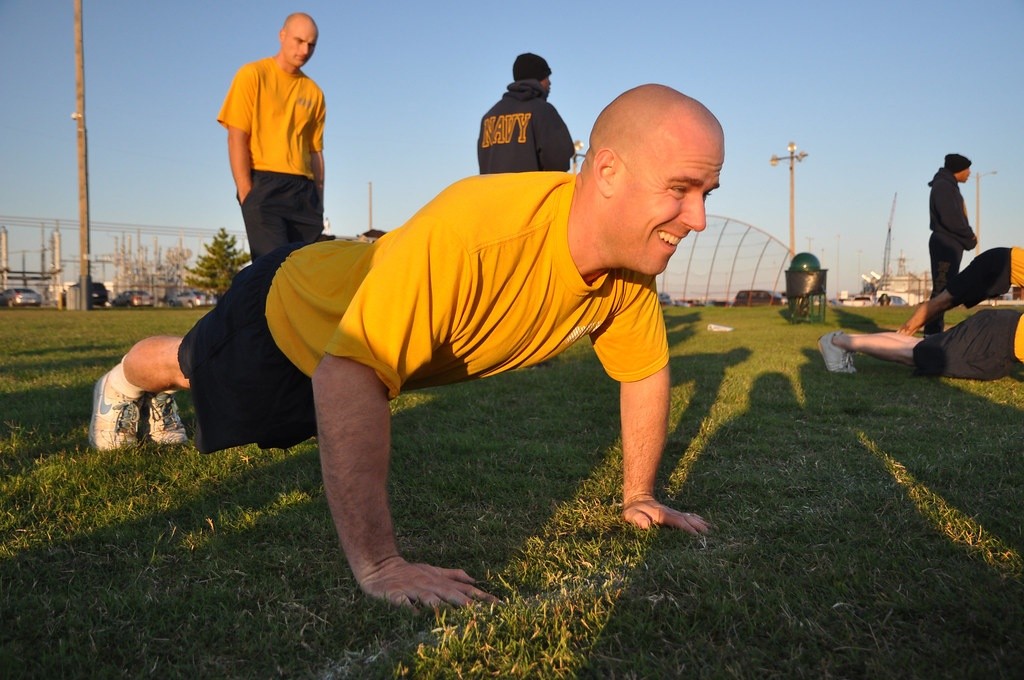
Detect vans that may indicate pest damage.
[733,289,789,307]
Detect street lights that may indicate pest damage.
[769,141,808,264]
[970,168,999,257]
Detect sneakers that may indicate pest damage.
[145,389,188,448]
[87,371,141,455]
[817,332,858,376]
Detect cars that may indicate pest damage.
[872,296,910,307]
[166,291,217,308]
[0,288,43,308]
[111,291,155,307]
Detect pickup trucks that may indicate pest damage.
[842,294,874,307]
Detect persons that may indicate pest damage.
[922,153,976,337]
[477,54,576,364]
[817,309,1024,381]
[216,11,327,264]
[895,245,1024,336]
[84,84,725,616]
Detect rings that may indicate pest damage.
[685,512,690,516]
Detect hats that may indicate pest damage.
[945,154,972,175]
[512,52,552,84]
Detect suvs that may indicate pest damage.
[656,293,733,308]
[60,282,109,308]
